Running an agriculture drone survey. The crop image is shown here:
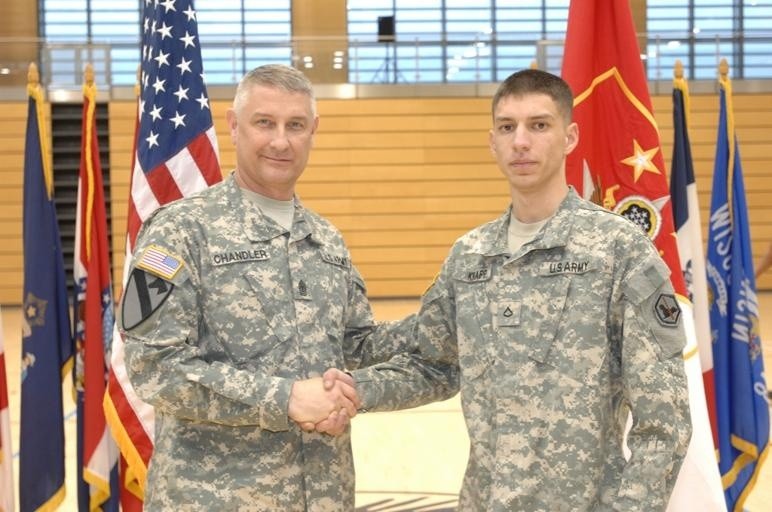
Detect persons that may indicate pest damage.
[301,66,695,512]
[122,63,421,511]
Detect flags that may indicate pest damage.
[16,60,75,512]
[1,335,19,511]
[666,59,720,466]
[103,0,227,512]
[556,1,729,512]
[708,59,771,512]
[75,65,121,511]
